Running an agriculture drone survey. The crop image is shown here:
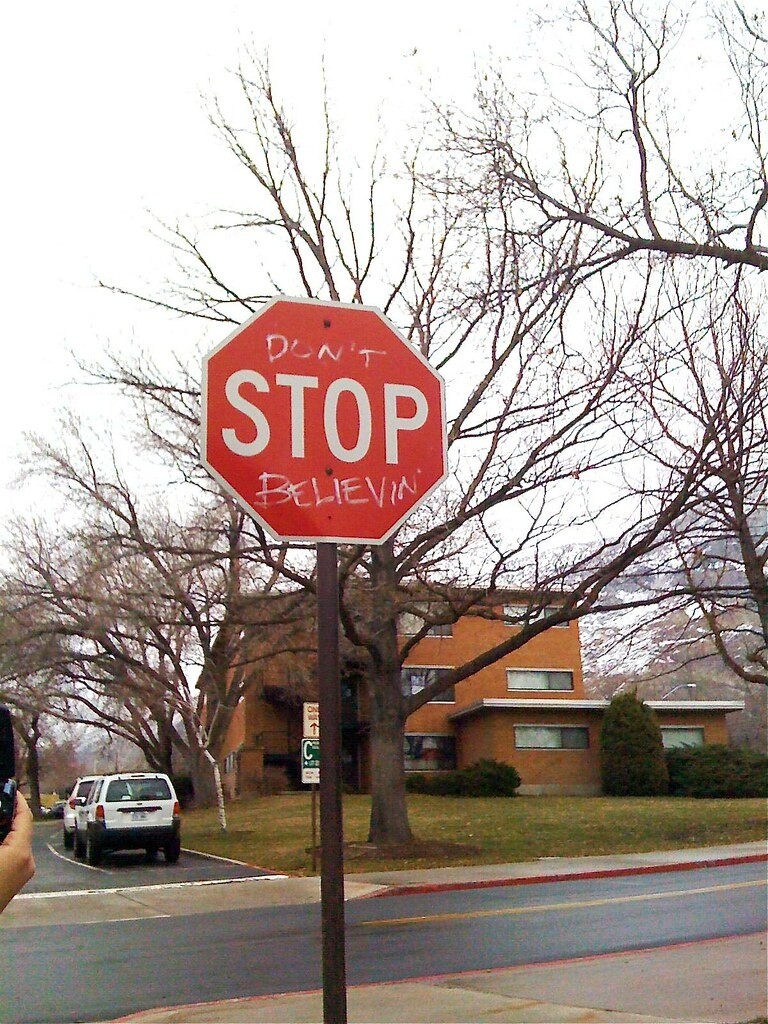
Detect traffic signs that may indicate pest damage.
[302,701,320,738]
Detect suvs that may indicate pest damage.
[53,770,181,865]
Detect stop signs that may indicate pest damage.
[199,295,449,545]
